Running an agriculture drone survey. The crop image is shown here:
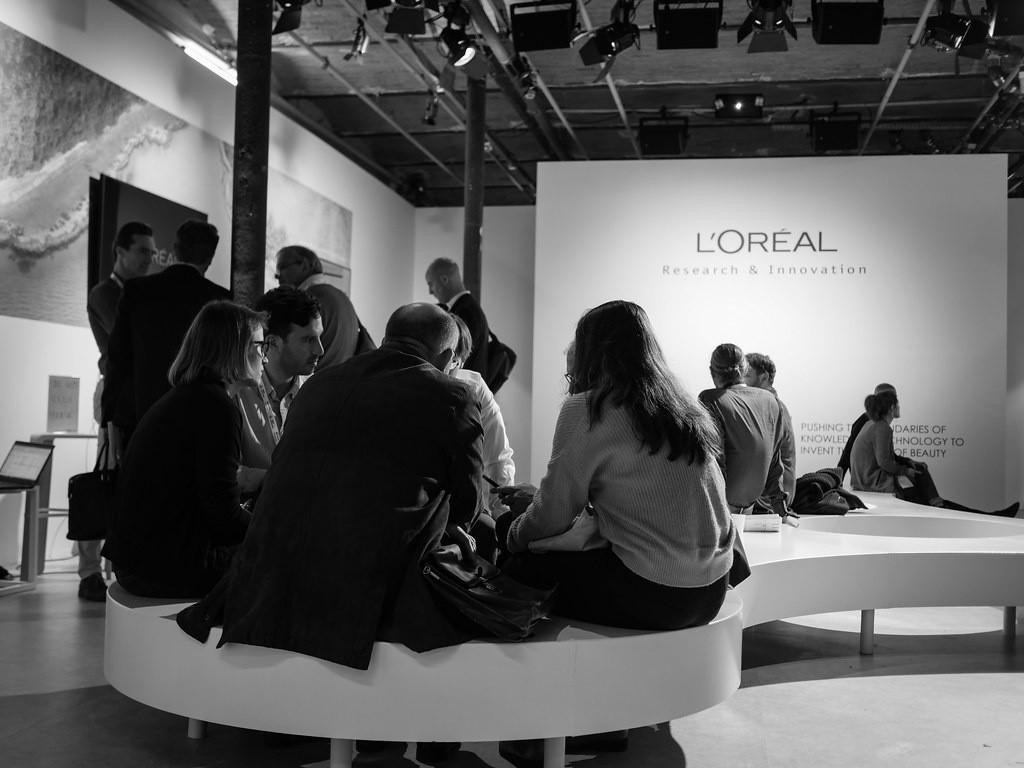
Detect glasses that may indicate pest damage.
[565,366,575,384]
[248,341,271,356]
[138,247,157,258]
[275,261,312,278]
[442,347,458,371]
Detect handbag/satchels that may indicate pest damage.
[418,534,555,643]
[353,315,378,356]
[67,437,125,541]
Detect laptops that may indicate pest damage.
[0,441,55,490]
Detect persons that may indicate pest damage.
[427,257,489,382]
[838,384,1019,516]
[109,286,733,768]
[744,353,796,515]
[72,220,229,603]
[273,245,359,373]
[697,342,798,528]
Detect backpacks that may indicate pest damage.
[487,331,516,396]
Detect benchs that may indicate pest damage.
[101,488,1024,768]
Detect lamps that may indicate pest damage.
[923,12,990,54]
[736,0,797,53]
[810,1,885,48]
[523,72,538,100]
[509,0,577,52]
[650,0,726,49]
[436,23,477,71]
[270,0,309,35]
[384,6,426,37]
[982,0,1024,38]
[711,91,764,119]
[804,109,862,151]
[636,114,692,154]
[579,0,643,81]
[421,100,437,127]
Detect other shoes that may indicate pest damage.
[79,573,106,601]
[417,742,462,766]
[994,502,1019,518]
[497,742,544,768]
[355,740,406,764]
[565,730,630,753]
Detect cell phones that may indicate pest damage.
[481,475,500,488]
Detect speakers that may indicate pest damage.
[811,2,884,45]
[656,7,721,50]
[513,9,576,52]
[639,125,687,157]
[812,120,859,151]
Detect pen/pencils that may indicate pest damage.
[482,475,499,487]
